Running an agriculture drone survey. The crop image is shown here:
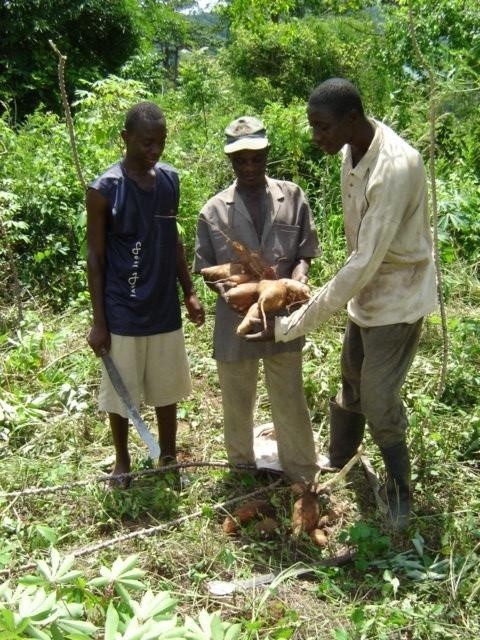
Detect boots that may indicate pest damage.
[327,397,366,470]
[379,440,415,528]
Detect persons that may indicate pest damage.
[85,102,206,490]
[238,78,438,534]
[191,116,324,488]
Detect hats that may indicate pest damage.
[224,116,269,155]
